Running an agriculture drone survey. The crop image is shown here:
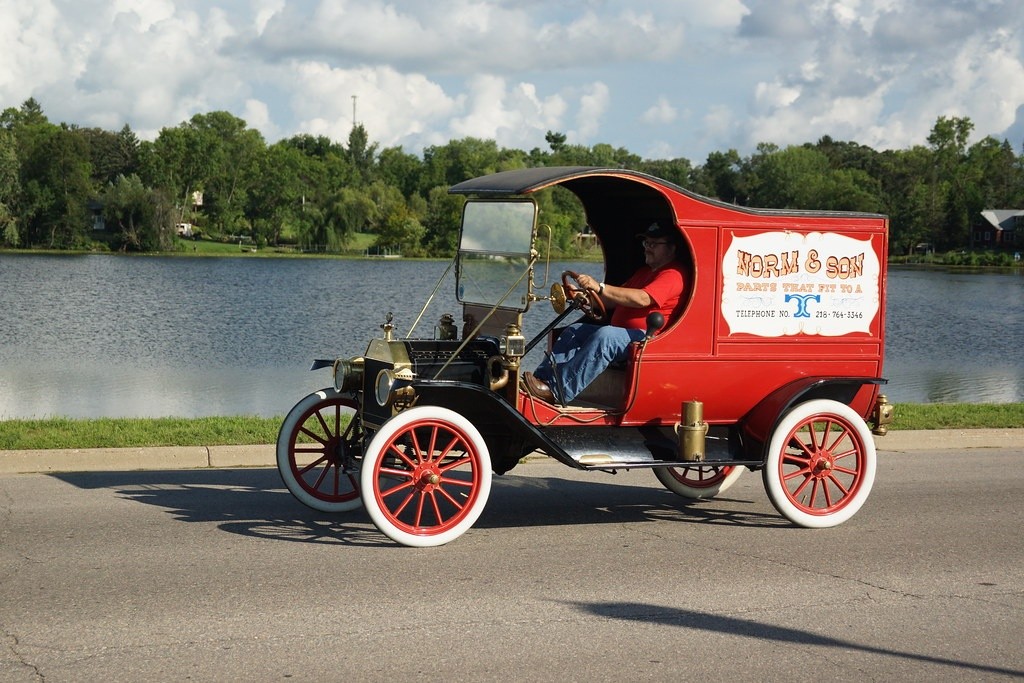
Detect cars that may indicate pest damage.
[276,166,890,548]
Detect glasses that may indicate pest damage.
[642,240,668,248]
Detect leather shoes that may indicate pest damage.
[523,371,554,403]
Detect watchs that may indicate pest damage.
[597,282,606,295]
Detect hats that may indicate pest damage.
[634,220,683,238]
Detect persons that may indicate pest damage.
[523,219,692,408]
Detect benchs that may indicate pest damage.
[571,367,630,409]
